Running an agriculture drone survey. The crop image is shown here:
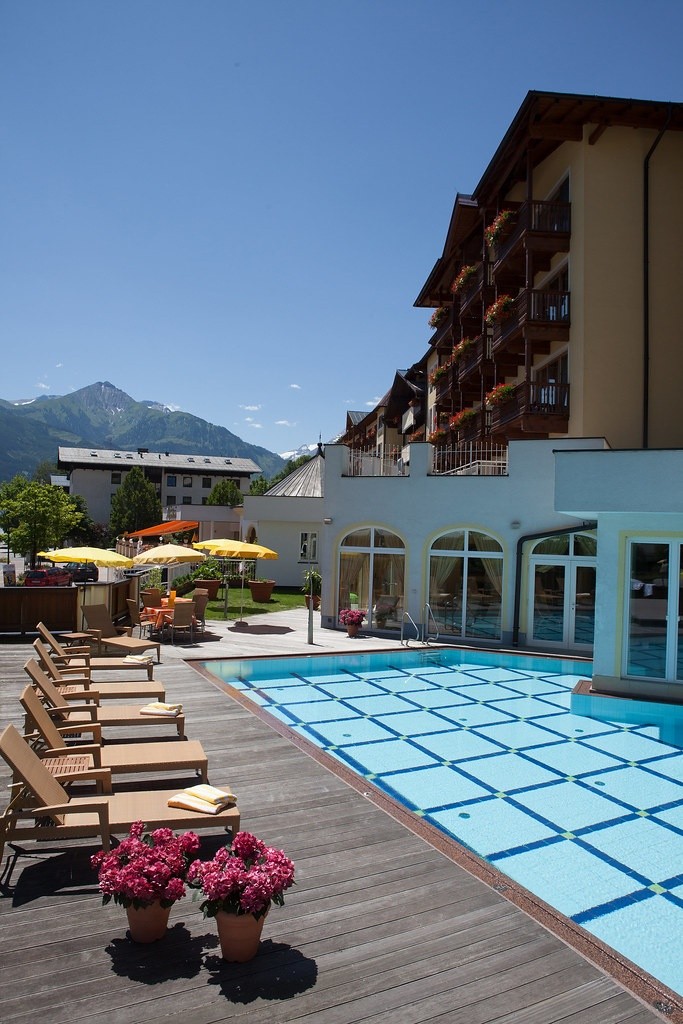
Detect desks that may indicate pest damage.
[36,686,78,708]
[59,632,94,647]
[40,757,89,775]
[161,597,193,610]
[140,605,194,629]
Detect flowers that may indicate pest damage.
[428,429,447,445]
[186,831,297,920]
[448,408,478,433]
[428,361,449,387]
[375,606,394,621]
[451,337,475,364]
[89,819,200,910]
[410,431,422,441]
[485,381,515,408]
[450,265,477,299]
[339,609,366,628]
[483,208,516,250]
[483,295,514,326]
[428,304,451,329]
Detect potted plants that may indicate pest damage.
[248,576,275,602]
[301,564,322,609]
[193,555,223,601]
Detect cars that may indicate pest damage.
[63,562,99,582]
[25,567,74,587]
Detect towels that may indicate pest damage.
[139,702,184,717]
[166,792,228,815]
[182,784,235,806]
[122,655,153,666]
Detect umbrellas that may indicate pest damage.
[209,539,280,625]
[132,542,206,597]
[193,539,244,601]
[35,546,134,633]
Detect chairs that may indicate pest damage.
[0,723,241,864]
[23,658,185,742]
[33,637,165,703]
[428,575,494,608]
[80,603,161,663]
[533,577,593,604]
[36,621,154,682]
[20,684,208,784]
[126,589,210,644]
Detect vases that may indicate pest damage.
[502,225,511,237]
[377,619,386,628]
[126,897,172,943]
[214,904,270,962]
[462,348,474,360]
[346,624,358,637]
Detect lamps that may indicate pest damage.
[324,518,332,524]
